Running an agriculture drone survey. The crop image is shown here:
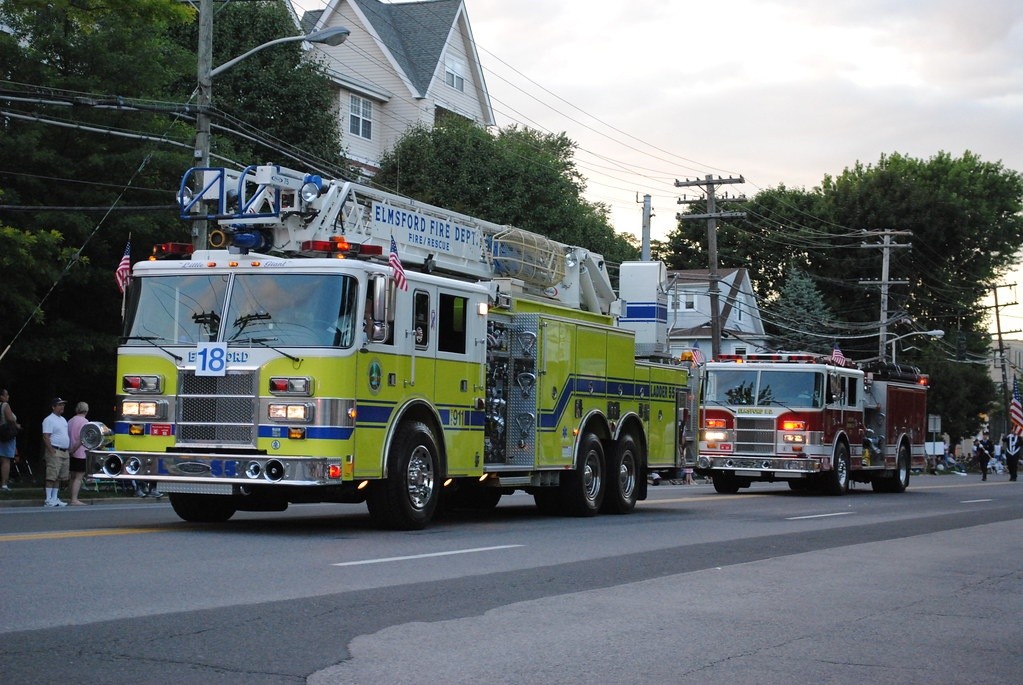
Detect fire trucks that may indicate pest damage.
[698,349,931,496]
[79,158,706,528]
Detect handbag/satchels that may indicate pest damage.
[0,423,17,442]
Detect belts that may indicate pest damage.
[52,445,69,452]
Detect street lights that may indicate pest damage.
[193,25,351,251]
[879,329,945,362]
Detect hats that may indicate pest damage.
[51,398,67,404]
[983,431,990,435]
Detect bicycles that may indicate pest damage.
[9,449,46,487]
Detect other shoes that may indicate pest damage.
[982,476,986,481]
[71,501,86,506]
[148,489,163,497]
[1009,476,1013,481]
[1013,476,1016,481]
[2,485,12,492]
[137,490,146,497]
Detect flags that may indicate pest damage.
[115,238,131,293]
[691,340,702,368]
[832,342,846,367]
[1009,373,1023,436]
[388,233,408,292]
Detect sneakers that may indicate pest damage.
[44,499,59,507]
[51,499,68,507]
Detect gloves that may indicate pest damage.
[979,444,983,448]
[985,450,989,454]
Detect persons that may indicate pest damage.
[126,480,164,498]
[42,397,70,507]
[0,389,23,491]
[326,292,375,345]
[947,428,1023,482]
[651,468,713,485]
[67,402,91,506]
